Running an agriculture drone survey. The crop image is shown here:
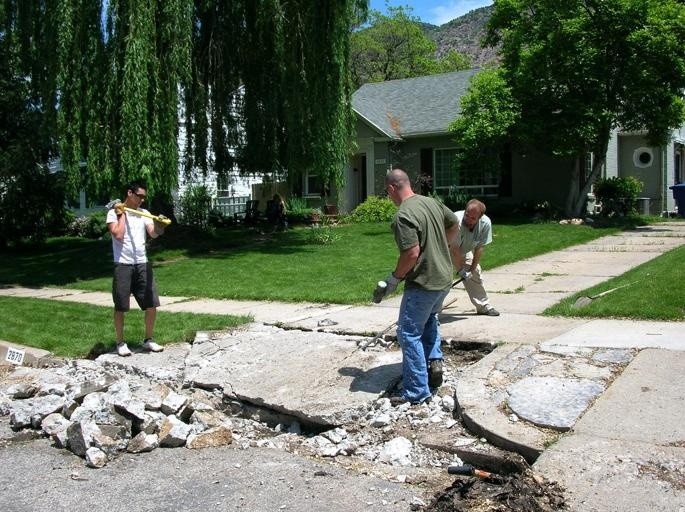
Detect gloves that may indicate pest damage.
[456,269,473,283]
[153,215,169,235]
[383,271,402,297]
[113,202,126,216]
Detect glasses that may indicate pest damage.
[133,191,147,200]
[383,183,395,196]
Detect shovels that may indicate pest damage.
[573,280,638,308]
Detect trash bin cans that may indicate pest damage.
[637,197,651,214]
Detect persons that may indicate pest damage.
[373,169,459,406]
[453,199,500,316]
[269,193,288,232]
[106,181,167,356]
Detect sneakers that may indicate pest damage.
[142,338,164,353]
[116,340,132,357]
[478,307,500,316]
[428,360,443,389]
[390,394,434,406]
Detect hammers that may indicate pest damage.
[371,278,408,302]
[105,199,171,225]
